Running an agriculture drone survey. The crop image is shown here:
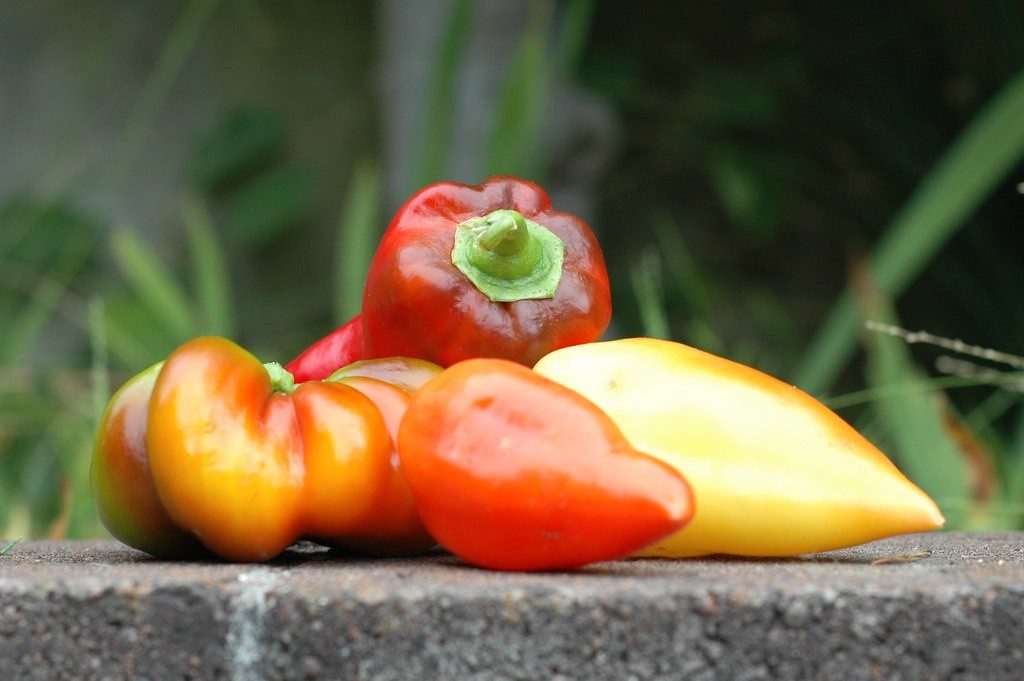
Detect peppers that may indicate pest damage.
[84,172,948,575]
